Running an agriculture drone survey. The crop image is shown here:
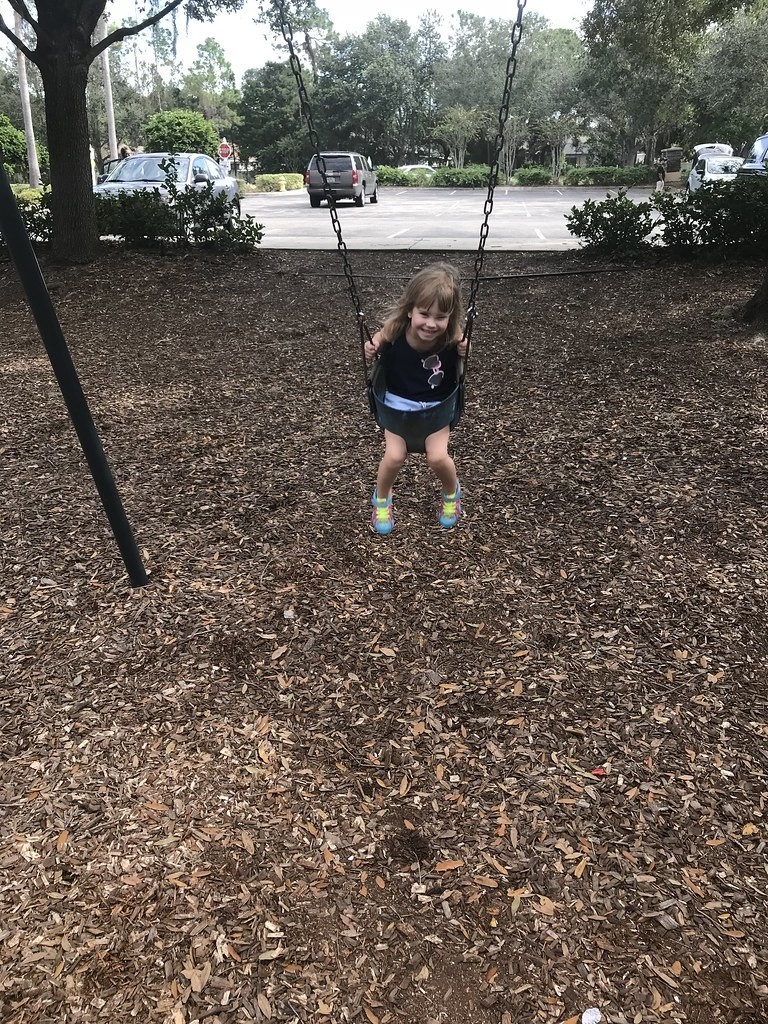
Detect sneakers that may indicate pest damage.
[439,480,461,528]
[372,488,394,534]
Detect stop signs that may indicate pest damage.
[218,143,232,159]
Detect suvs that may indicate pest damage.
[685,144,745,205]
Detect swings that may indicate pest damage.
[275,0,526,453]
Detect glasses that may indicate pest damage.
[421,354,444,389]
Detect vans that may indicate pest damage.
[305,151,378,207]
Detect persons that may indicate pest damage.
[363,263,472,535]
[117,146,131,165]
[656,157,668,193]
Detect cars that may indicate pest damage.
[736,131,768,178]
[399,165,456,181]
[93,153,241,235]
